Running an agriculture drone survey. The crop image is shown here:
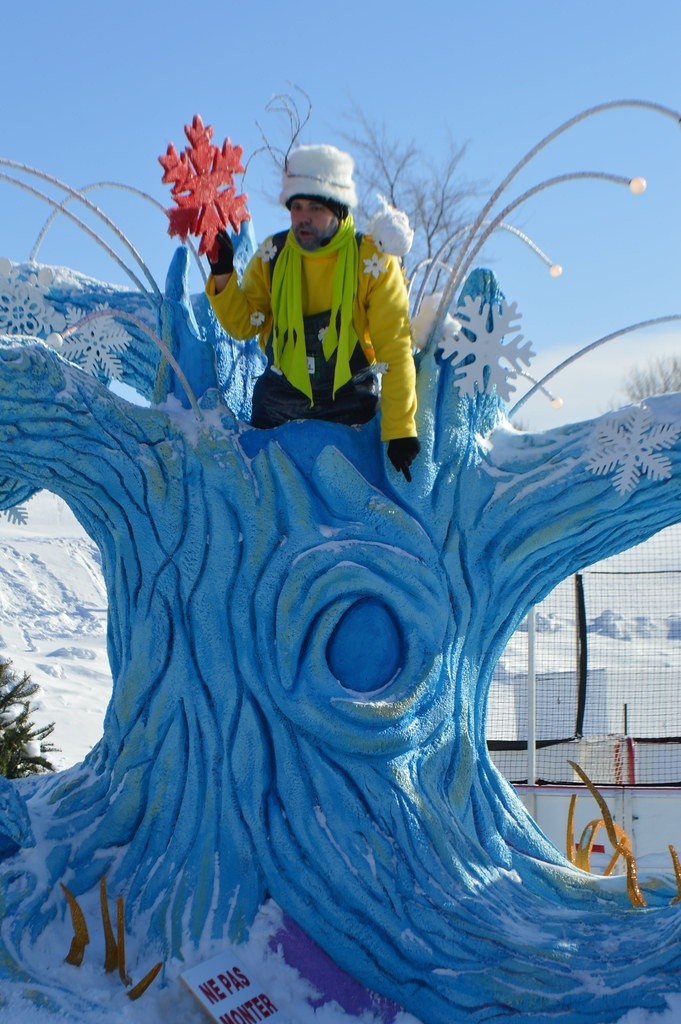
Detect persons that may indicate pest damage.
[199,144,422,484]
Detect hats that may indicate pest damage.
[277,144,358,207]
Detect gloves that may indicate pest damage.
[387,437,420,483]
[206,229,234,275]
